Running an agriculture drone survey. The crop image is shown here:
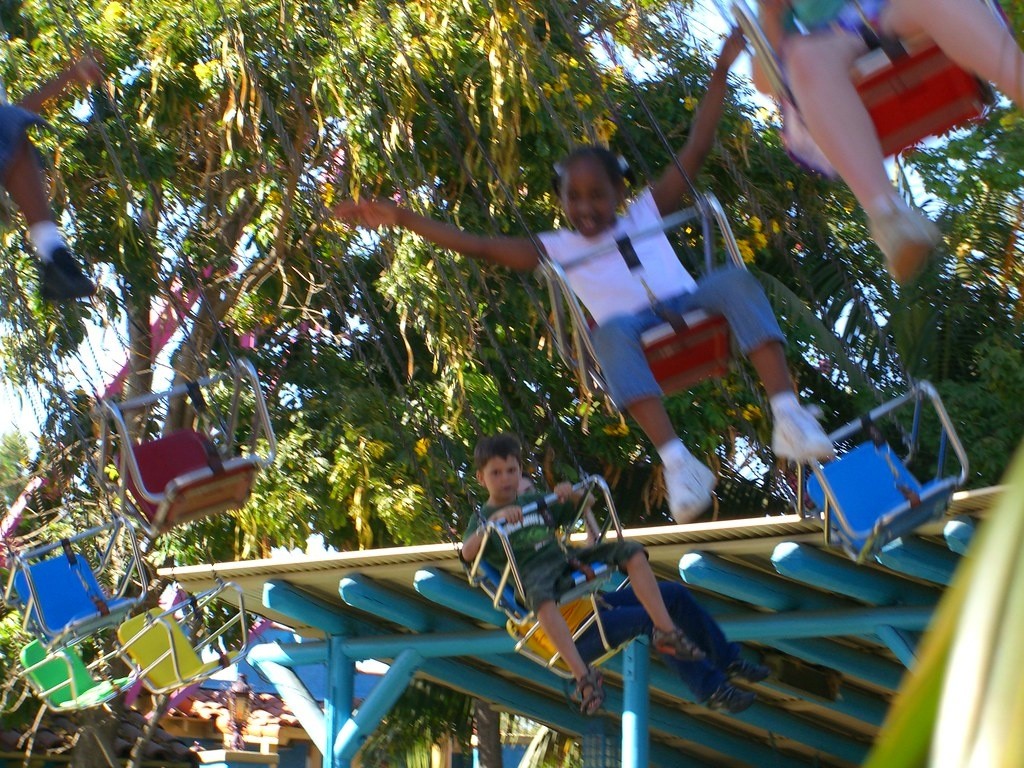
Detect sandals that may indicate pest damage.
[651,626,708,664]
[572,662,605,716]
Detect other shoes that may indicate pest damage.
[33,246,98,299]
[866,205,943,284]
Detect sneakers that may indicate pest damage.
[723,649,771,683]
[770,405,836,465]
[662,453,718,525]
[706,679,759,714]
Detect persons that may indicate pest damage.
[751,0,1024,286]
[334,23,840,527]
[1,48,106,298]
[459,431,775,719]
